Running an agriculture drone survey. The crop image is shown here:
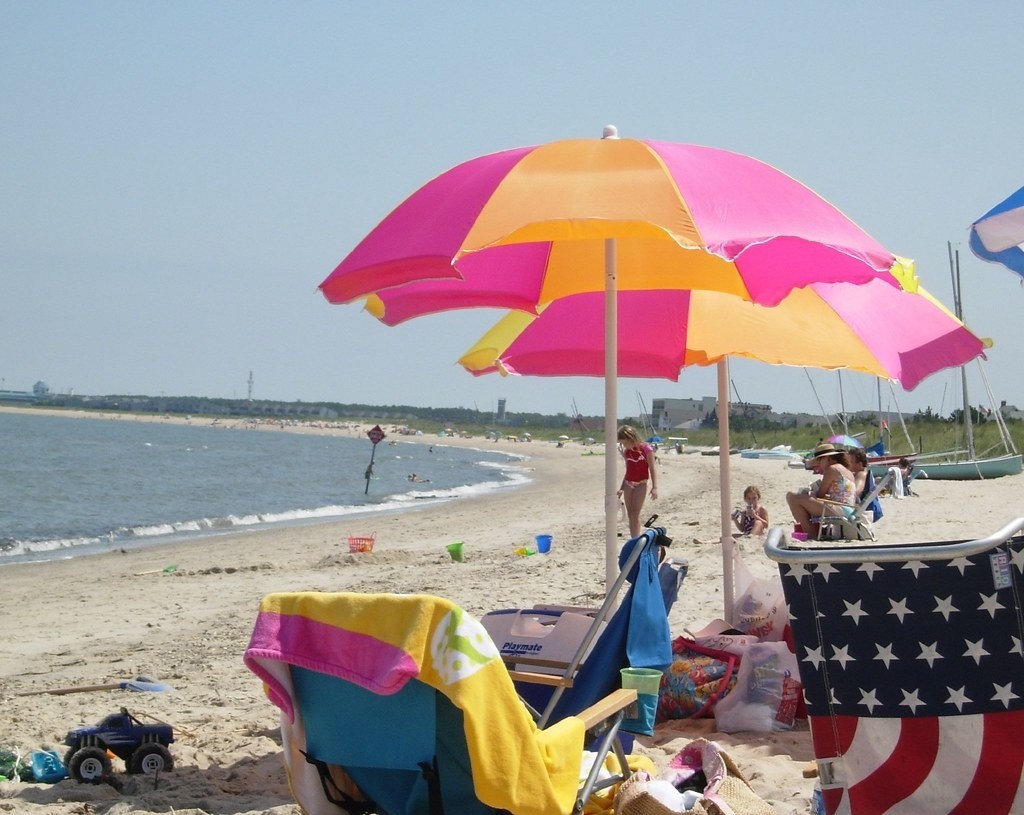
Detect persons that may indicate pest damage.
[408,473,430,483]
[849,449,883,523]
[365,460,376,480]
[693,486,768,544]
[429,446,433,453]
[786,444,856,538]
[875,458,909,496]
[617,425,658,539]
[817,438,824,446]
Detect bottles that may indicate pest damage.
[810,779,826,815]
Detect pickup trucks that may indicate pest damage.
[62,709,174,784]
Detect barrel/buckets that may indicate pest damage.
[447,541,464,563]
[536,535,552,554]
[620,667,663,695]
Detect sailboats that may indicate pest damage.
[803,240,1023,481]
[634,391,662,445]
[730,378,797,460]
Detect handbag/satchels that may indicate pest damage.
[715,640,801,734]
[731,551,790,643]
[831,510,873,539]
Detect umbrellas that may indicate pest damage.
[312,125,994,634]
[644,434,663,442]
[967,186,1024,286]
[826,434,865,449]
[559,435,569,439]
[585,437,595,446]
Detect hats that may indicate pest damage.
[805,443,846,460]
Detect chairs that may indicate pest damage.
[765,527,1024,815]
[243,527,689,815]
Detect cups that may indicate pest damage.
[746,506,753,517]
